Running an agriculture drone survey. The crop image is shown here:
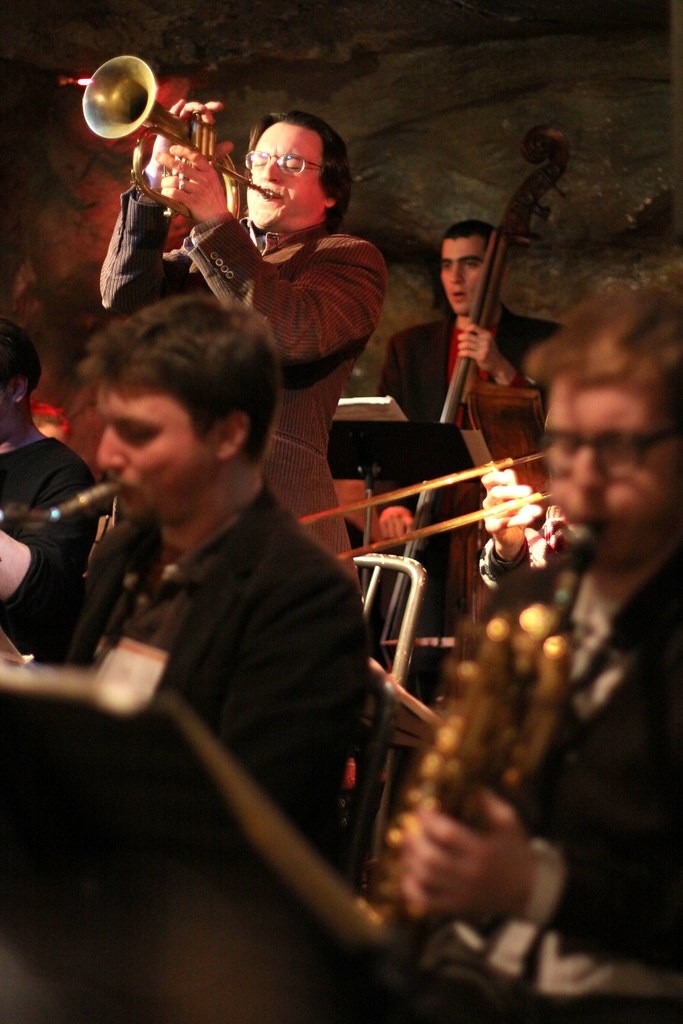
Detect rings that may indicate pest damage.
[471,335,476,344]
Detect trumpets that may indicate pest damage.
[80,52,283,225]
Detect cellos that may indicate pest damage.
[379,124,575,677]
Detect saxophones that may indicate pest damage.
[365,510,604,942]
[50,478,121,520]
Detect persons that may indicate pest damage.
[0,294,366,1024]
[376,218,563,697]
[98,97,386,574]
[378,288,683,1024]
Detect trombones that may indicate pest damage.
[300,449,552,564]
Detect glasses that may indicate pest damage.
[244,151,325,175]
[541,426,683,477]
